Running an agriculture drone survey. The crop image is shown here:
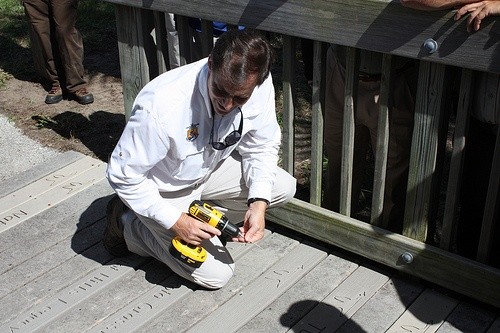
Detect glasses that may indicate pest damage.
[211,106,244,149]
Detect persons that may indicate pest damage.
[22,0,93,104]
[101,28,297,290]
[323,0,500,257]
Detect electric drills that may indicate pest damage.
[169,200,263,268]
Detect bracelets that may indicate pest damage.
[248,198,270,206]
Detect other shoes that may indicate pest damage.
[46,84,66,103]
[102,196,128,256]
[73,87,94,103]
